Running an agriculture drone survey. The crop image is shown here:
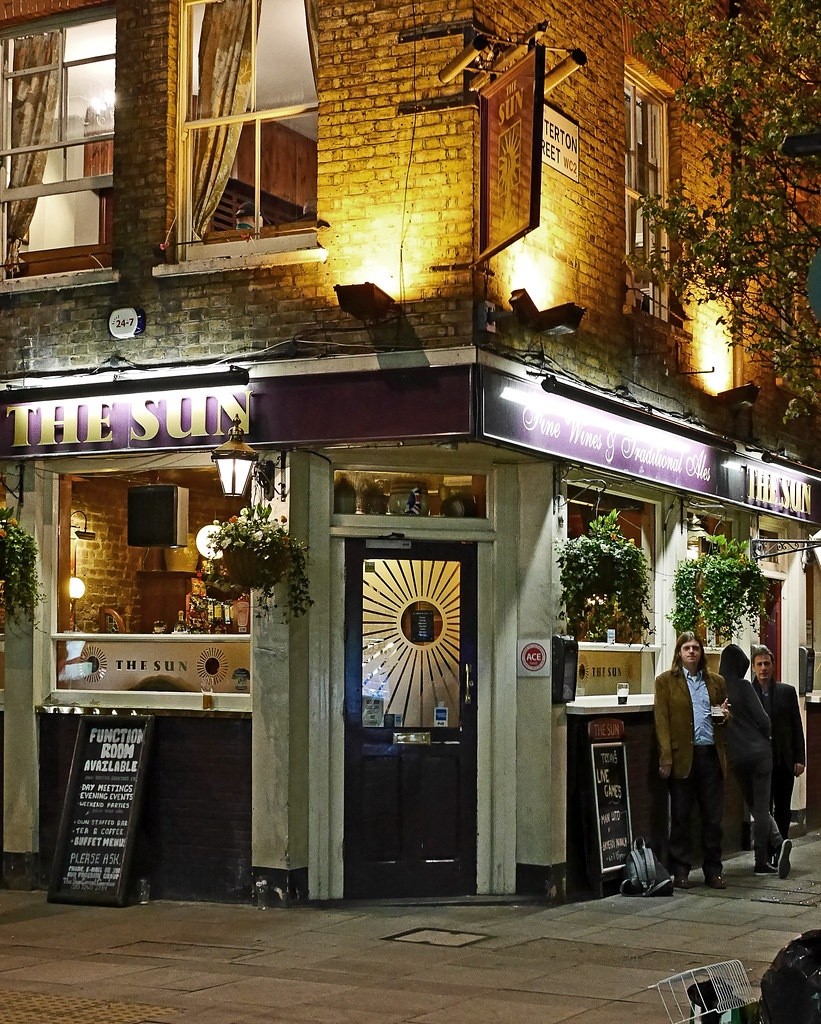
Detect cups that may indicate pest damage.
[711,705,724,725]
[617,683,629,705]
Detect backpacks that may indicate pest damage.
[620,837,675,897]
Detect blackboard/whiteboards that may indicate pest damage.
[590,743,638,873]
[46,714,157,907]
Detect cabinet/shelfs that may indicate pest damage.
[135,570,250,634]
[82,95,319,244]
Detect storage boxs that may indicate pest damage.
[689,1001,759,1024]
[647,959,758,1024]
[127,485,189,549]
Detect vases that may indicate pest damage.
[222,547,288,590]
[164,532,199,571]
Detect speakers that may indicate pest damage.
[127,484,188,548]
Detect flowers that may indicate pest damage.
[0,505,49,638]
[203,501,317,627]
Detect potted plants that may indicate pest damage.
[553,507,658,656]
[663,533,779,652]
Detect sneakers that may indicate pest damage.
[753,862,778,876]
[778,839,793,879]
[674,875,689,889]
[704,873,727,889]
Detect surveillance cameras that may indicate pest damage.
[508,288,542,328]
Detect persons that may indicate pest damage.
[654,631,732,889]
[717,645,805,879]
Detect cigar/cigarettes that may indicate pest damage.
[722,703,731,707]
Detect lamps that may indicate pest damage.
[0,359,250,404]
[745,447,821,482]
[538,301,587,337]
[195,518,224,561]
[485,289,540,334]
[716,381,762,410]
[70,511,97,542]
[332,283,395,322]
[70,577,86,633]
[526,370,738,454]
[210,413,286,504]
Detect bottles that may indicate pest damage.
[173,611,186,632]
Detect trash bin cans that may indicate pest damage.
[762,929,821,1024]
